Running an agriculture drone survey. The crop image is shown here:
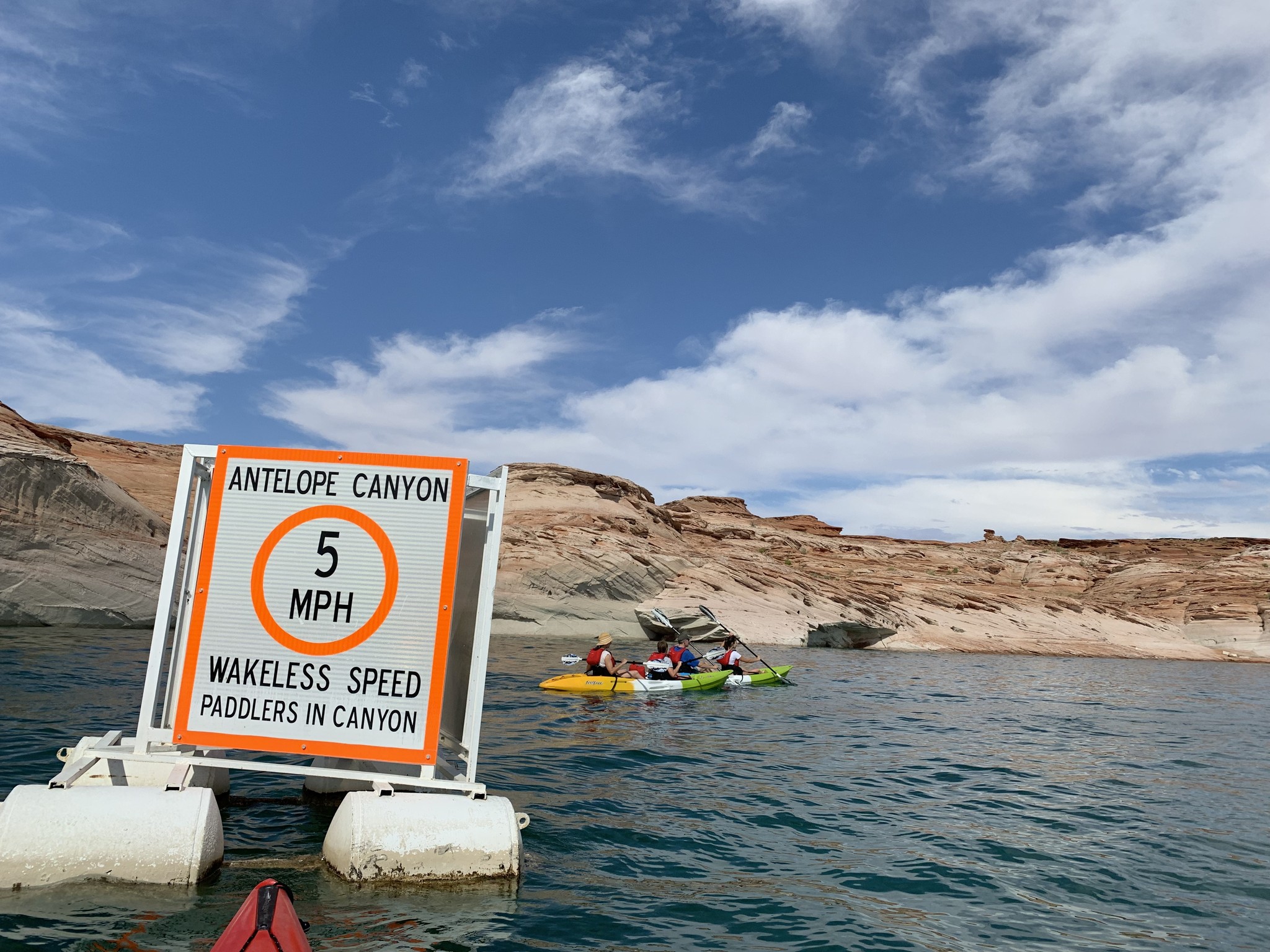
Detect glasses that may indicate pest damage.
[686,639,690,642]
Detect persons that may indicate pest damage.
[669,633,719,674]
[648,641,691,681]
[717,632,766,675]
[586,633,645,679]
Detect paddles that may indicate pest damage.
[652,606,744,685]
[699,605,793,685]
[673,646,725,666]
[562,654,670,672]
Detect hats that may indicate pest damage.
[596,633,614,645]
[678,633,692,640]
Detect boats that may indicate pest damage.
[668,663,794,690]
[538,668,735,693]
[207,876,313,952]
